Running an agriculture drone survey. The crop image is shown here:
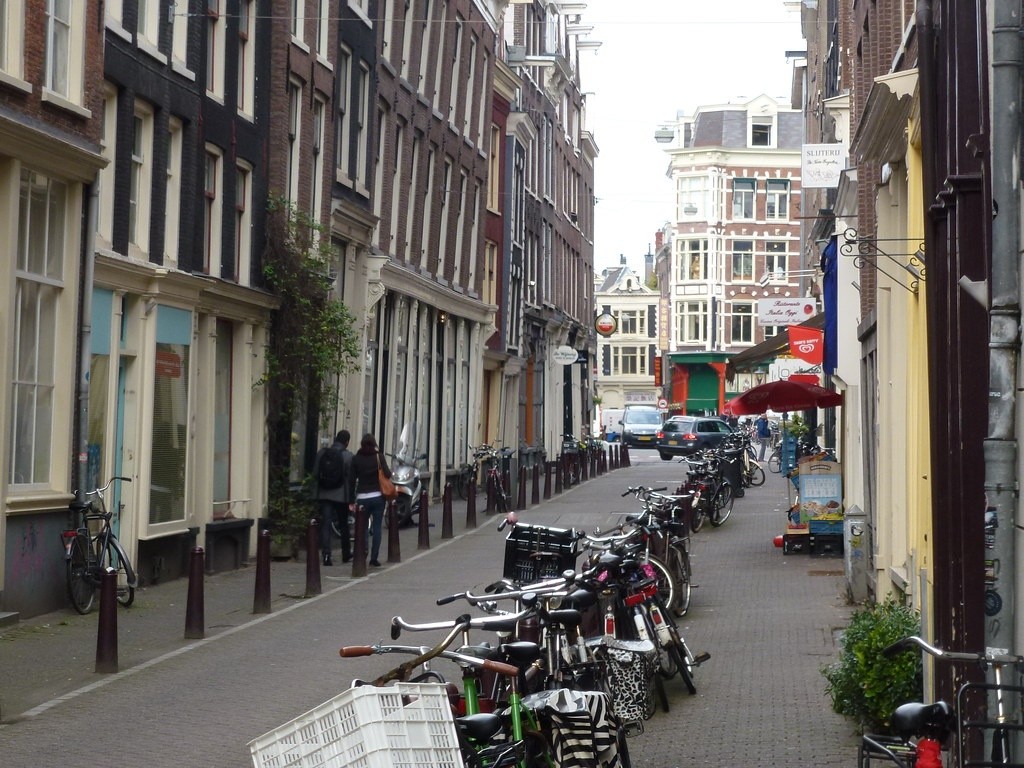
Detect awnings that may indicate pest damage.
[725,311,825,386]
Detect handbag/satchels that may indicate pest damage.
[585,635,661,720]
[501,688,624,768]
[376,453,399,502]
[562,442,580,454]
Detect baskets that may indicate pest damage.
[84,497,103,536]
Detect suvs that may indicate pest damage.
[618,404,664,448]
[656,416,734,460]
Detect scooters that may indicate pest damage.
[385,420,431,527]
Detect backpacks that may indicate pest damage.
[315,447,347,490]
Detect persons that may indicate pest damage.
[757,413,771,462]
[310,430,359,566]
[720,414,738,428]
[149,421,181,523]
[349,433,392,567]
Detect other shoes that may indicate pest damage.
[323,554,333,566]
[758,459,767,462]
[369,560,381,567]
[342,551,354,563]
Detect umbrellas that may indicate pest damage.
[723,378,843,508]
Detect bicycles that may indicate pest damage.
[477,450,513,512]
[768,439,812,473]
[59,476,138,614]
[330,477,374,540]
[339,424,766,768]
[856,635,1024,768]
[458,444,499,500]
